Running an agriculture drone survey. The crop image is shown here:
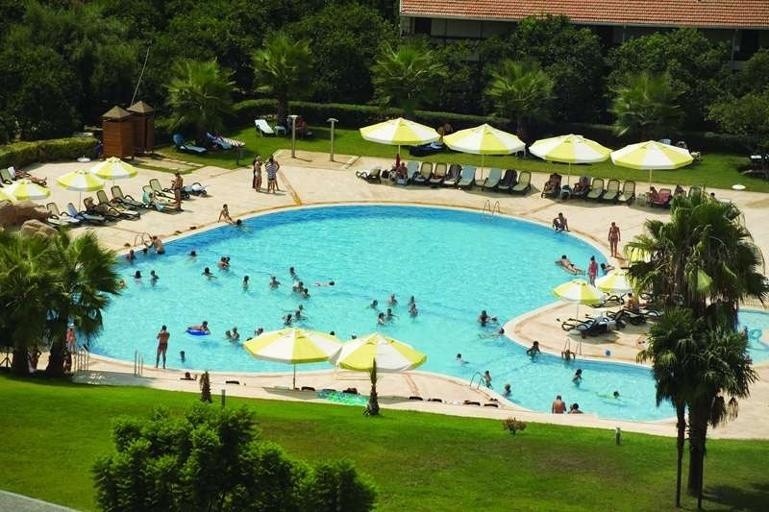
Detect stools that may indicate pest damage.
[275,125,287,136]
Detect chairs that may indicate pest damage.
[650,186,701,209]
[173,130,233,155]
[540,172,636,206]
[355,160,532,194]
[46,178,180,227]
[0,166,46,188]
[562,292,665,339]
[254,118,274,137]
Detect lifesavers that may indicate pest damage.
[188,326,210,335]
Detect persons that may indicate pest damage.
[88,196,116,214]
[396,162,408,179]
[180,371,195,380]
[153,325,171,369]
[675,185,683,195]
[13,170,47,186]
[148,193,179,210]
[66,321,77,355]
[555,254,582,275]
[252,155,262,188]
[608,221,621,260]
[649,187,660,207]
[254,156,262,191]
[552,212,571,235]
[576,176,589,194]
[265,155,281,191]
[126,235,418,342]
[170,171,183,214]
[179,350,186,361]
[545,172,559,194]
[586,254,599,289]
[266,158,276,194]
[216,204,242,228]
[623,292,639,313]
[600,262,615,275]
[457,311,619,416]
[18,168,48,183]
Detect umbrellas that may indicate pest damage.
[608,141,695,190]
[360,117,443,157]
[594,266,639,311]
[331,331,429,374]
[55,167,105,213]
[243,325,341,388]
[442,122,527,182]
[90,156,138,188]
[529,132,613,190]
[554,277,606,330]
[0,176,52,208]
